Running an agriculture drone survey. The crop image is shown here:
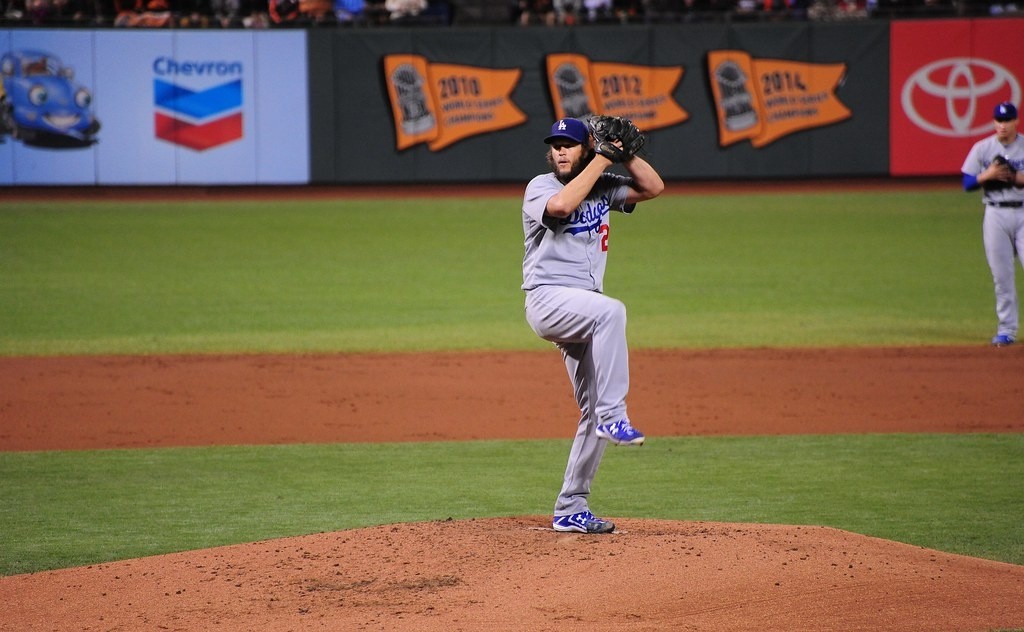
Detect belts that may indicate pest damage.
[990,202,1023,206]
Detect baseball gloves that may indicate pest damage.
[586,114,646,164]
[990,155,1018,190]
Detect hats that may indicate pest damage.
[544,118,589,144]
[994,104,1016,118]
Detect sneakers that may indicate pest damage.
[596,420,645,446]
[993,335,1013,345]
[553,510,615,533]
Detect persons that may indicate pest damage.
[0,0,1024,28]
[961,101,1024,347]
[521,115,665,534]
